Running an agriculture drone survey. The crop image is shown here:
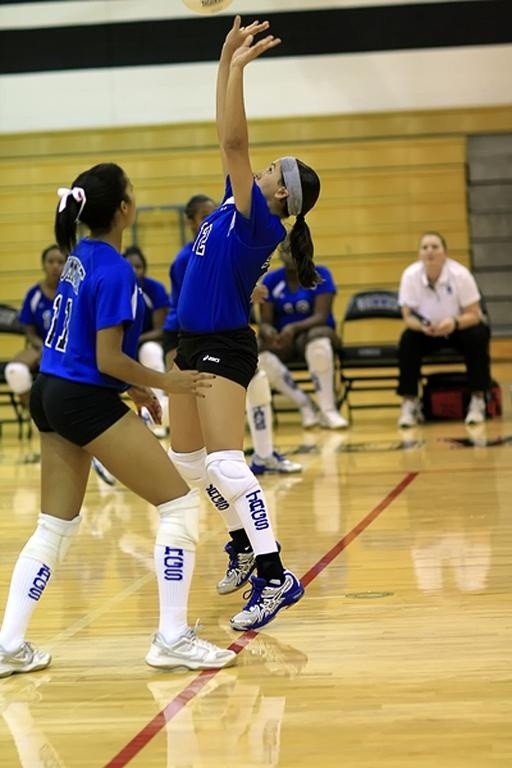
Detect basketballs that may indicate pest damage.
[185,0,234,14]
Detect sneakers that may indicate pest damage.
[142,617,242,670]
[0,640,55,680]
[248,450,305,474]
[90,455,117,490]
[215,537,283,597]
[227,570,307,633]
[396,399,418,427]
[297,404,318,428]
[319,411,348,429]
[463,393,486,427]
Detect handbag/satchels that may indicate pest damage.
[422,371,504,423]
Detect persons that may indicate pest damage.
[0,161,239,677]
[164,12,323,635]
[259,229,348,430]
[394,231,490,426]
[5,194,302,485]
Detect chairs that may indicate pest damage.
[1,300,32,443]
[269,289,491,427]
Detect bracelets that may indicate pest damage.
[453,316,460,331]
